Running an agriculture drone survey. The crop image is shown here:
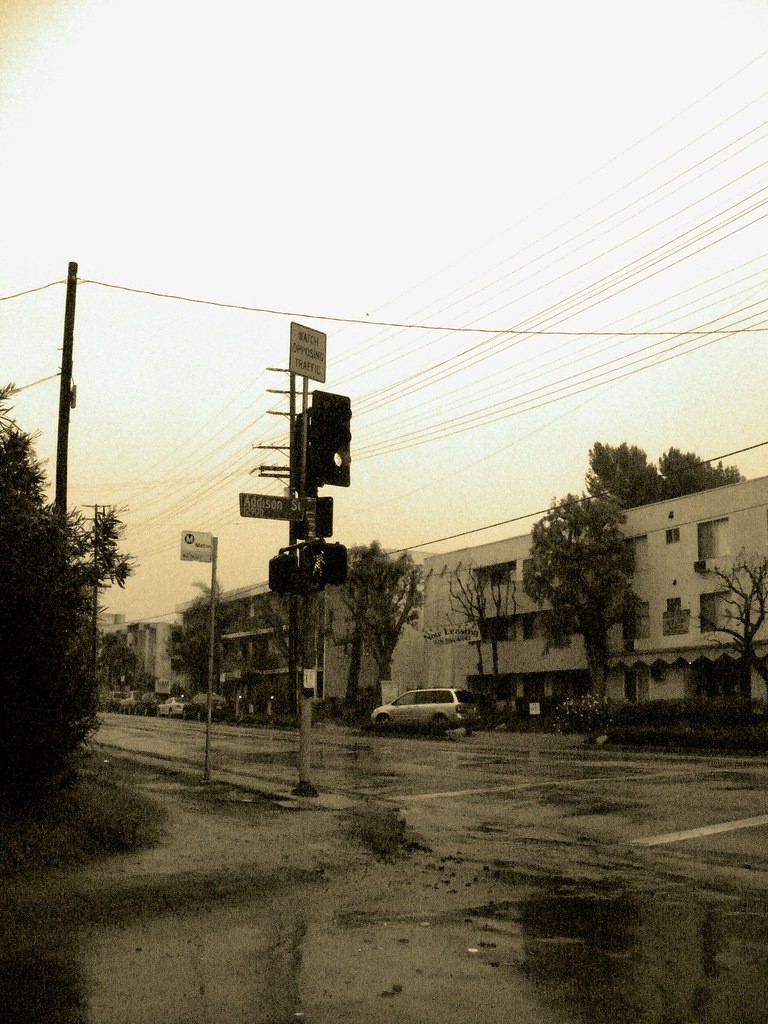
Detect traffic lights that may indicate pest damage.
[299,541,348,585]
[268,555,293,593]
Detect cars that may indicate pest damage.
[106,691,229,720]
[371,688,480,732]
